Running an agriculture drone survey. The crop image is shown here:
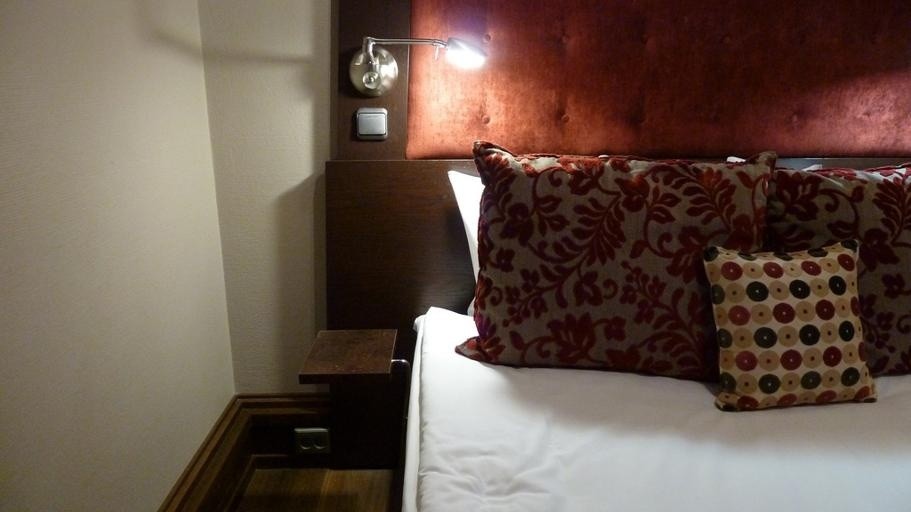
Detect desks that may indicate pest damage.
[299,328,412,421]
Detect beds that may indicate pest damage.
[402,304,911,512]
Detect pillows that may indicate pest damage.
[455,142,778,383]
[766,163,911,376]
[449,168,488,317]
[702,238,878,409]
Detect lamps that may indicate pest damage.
[349,37,491,99]
